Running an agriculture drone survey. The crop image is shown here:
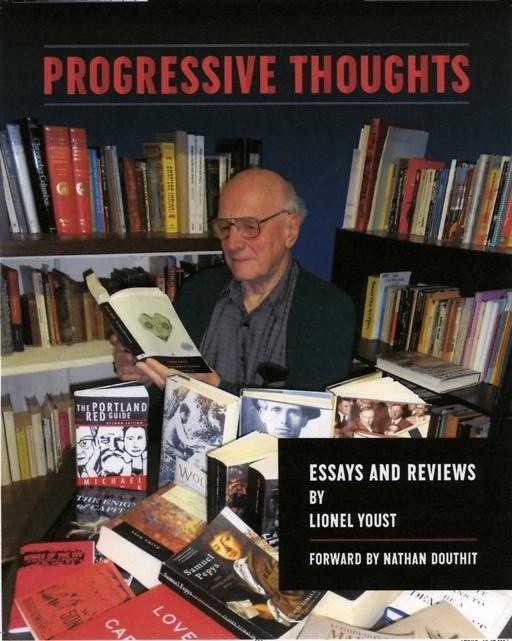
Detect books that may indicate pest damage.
[0,113,512,641]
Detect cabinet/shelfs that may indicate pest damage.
[0,234,265,563]
[328,228,511,438]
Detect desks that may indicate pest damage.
[0,469,511,640]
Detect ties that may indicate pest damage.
[341,415,346,426]
[392,421,395,425]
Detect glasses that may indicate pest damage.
[209,210,290,240]
[76,439,93,449]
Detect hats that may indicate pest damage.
[251,398,321,420]
